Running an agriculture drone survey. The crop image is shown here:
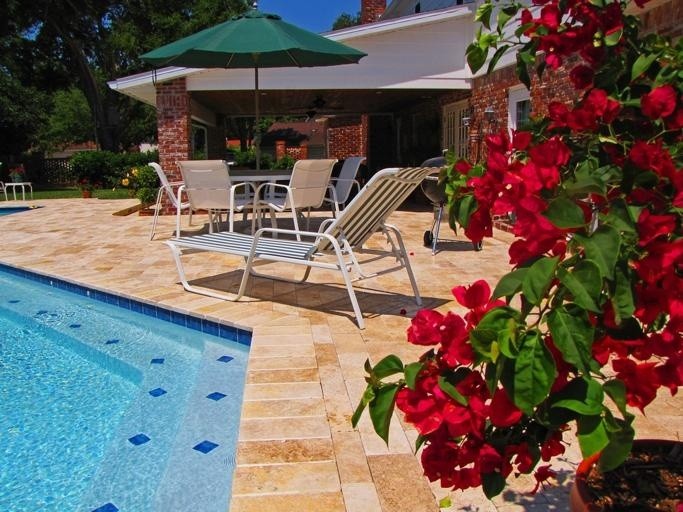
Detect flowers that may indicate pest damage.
[351,0,683,495]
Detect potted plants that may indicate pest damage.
[68,151,104,198]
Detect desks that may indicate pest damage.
[4,182,34,201]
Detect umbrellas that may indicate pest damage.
[137,1,368,199]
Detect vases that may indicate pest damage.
[570,440,682,512]
